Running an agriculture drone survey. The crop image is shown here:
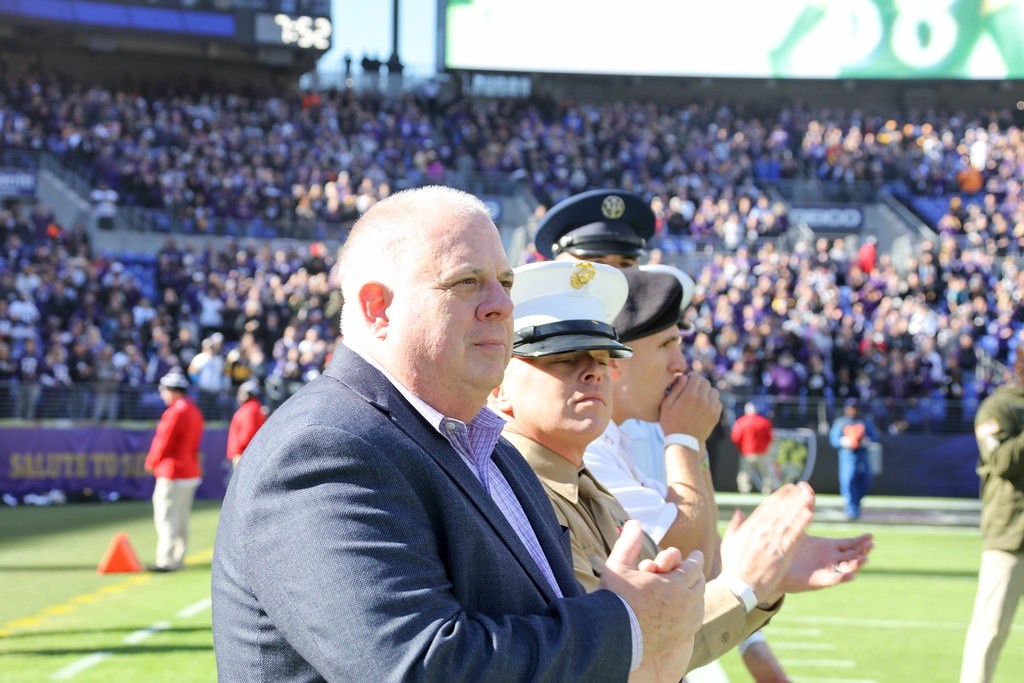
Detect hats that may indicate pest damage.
[843,397,859,407]
[500,260,633,359]
[532,188,656,256]
[240,381,260,397]
[160,373,189,388]
[610,262,696,341]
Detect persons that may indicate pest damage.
[0,65,1024,683]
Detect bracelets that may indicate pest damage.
[716,570,758,612]
[699,451,710,470]
[661,431,700,453]
